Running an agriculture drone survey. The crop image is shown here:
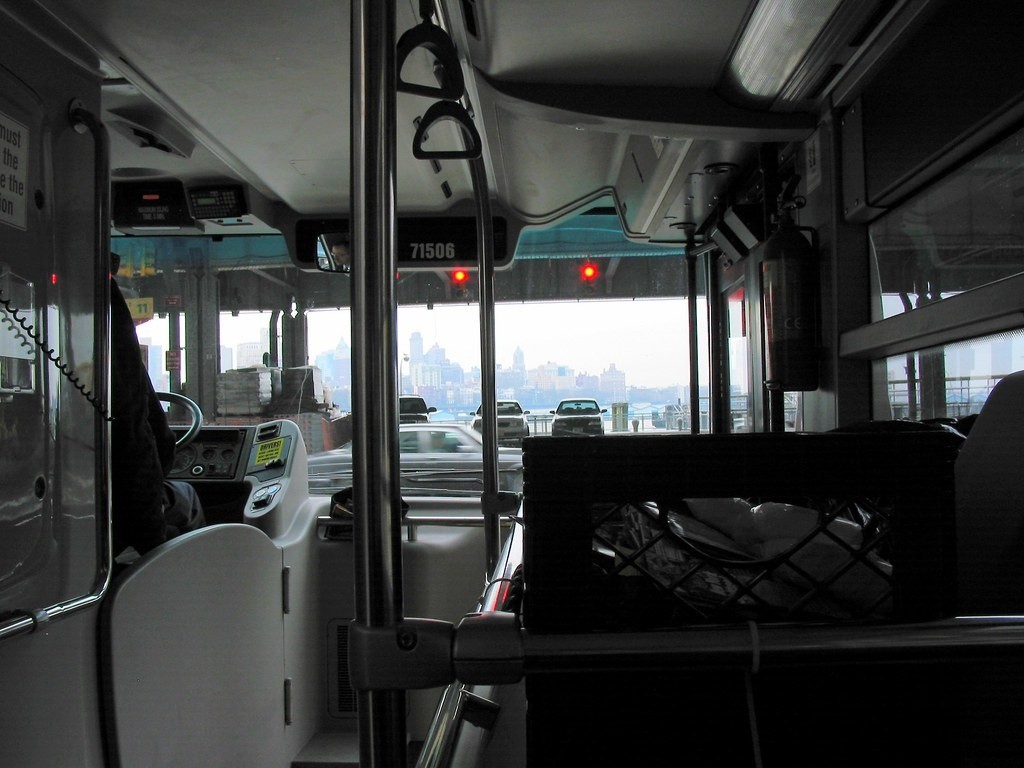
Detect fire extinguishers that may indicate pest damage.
[761,171,821,393]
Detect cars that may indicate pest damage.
[469,400,530,443]
[548,398,608,437]
[399,396,438,424]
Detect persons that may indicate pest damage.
[110,275,203,557]
